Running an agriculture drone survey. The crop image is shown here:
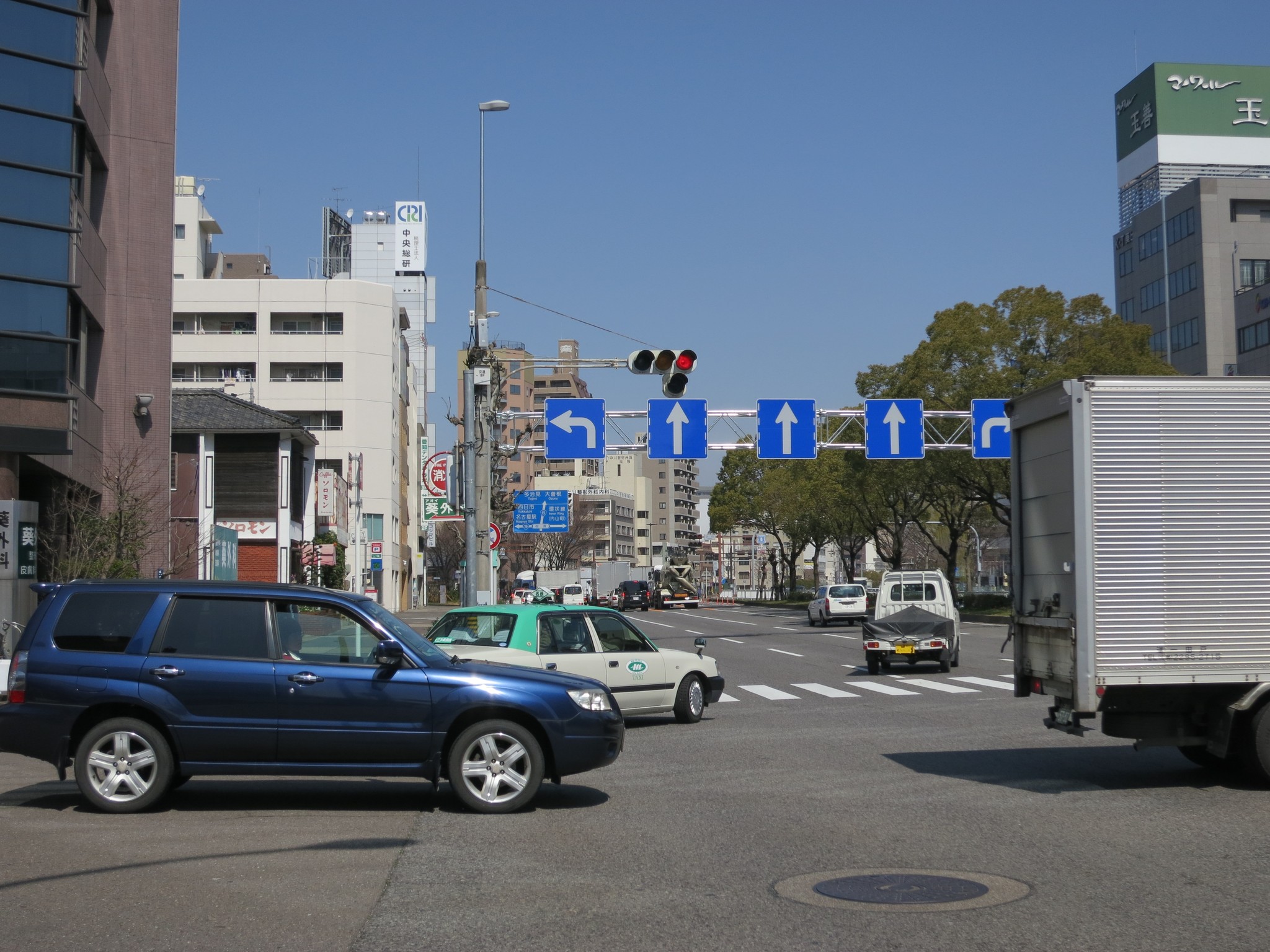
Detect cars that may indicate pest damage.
[607,588,620,609]
[785,586,810,593]
[419,603,725,722]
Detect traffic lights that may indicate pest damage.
[688,534,703,546]
[627,350,697,397]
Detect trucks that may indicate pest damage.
[1001,373,1270,792]
[852,576,873,588]
[511,560,656,600]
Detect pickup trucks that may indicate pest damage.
[862,568,965,671]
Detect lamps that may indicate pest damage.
[133,394,155,417]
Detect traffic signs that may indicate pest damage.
[513,489,568,532]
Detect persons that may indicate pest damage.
[278,618,303,661]
[539,621,551,653]
[592,621,620,652]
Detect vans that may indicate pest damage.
[508,584,584,609]
[618,580,650,612]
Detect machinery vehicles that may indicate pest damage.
[653,547,699,609]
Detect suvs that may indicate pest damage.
[807,584,868,626]
[0,572,626,812]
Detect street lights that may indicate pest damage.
[473,100,512,605]
